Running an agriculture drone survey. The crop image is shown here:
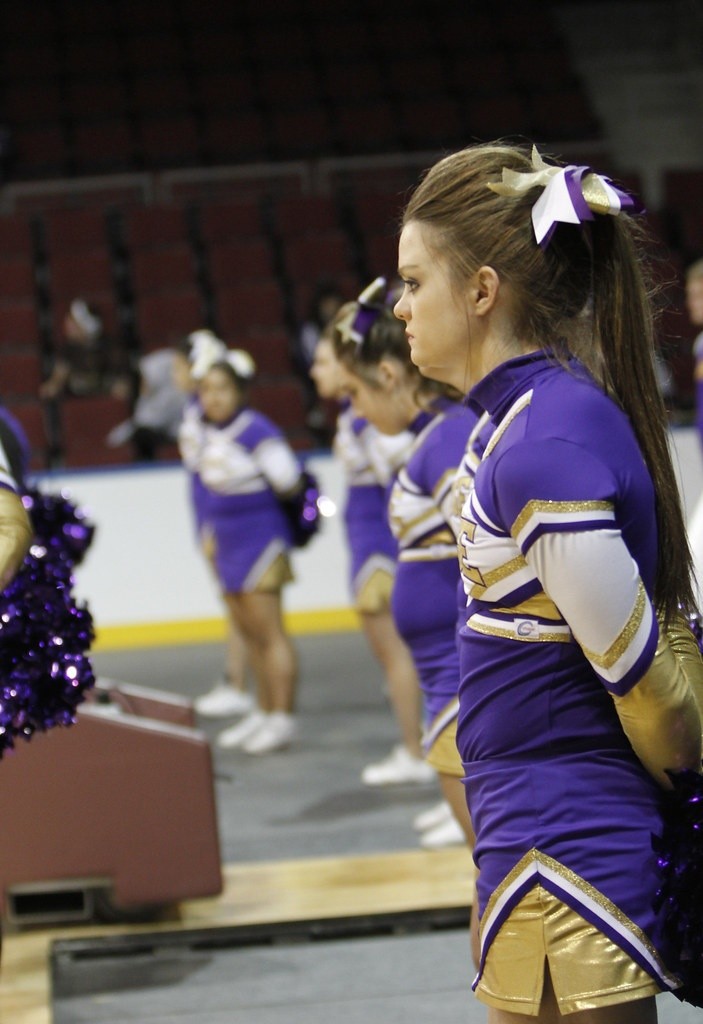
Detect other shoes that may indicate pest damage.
[217,709,298,752]
[195,684,255,716]
[361,749,435,786]
[412,803,465,847]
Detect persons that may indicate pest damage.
[392,146,703,1024]
[0,283,482,978]
[685,259,703,450]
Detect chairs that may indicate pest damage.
[0,0,703,468]
